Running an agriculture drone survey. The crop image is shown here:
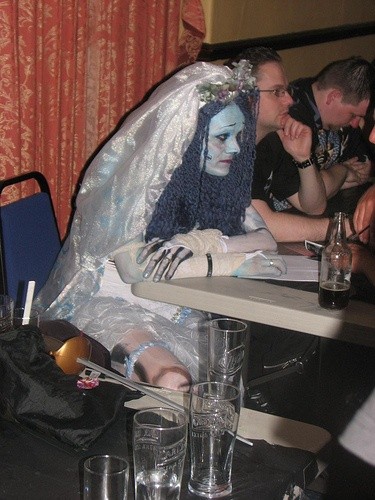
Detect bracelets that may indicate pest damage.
[205,253,213,277]
[294,156,314,168]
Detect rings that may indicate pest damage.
[270,259,273,266]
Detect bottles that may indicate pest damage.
[317,212,353,310]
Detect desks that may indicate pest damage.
[124,389,332,454]
[132,272,375,398]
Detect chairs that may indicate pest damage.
[0,171,61,311]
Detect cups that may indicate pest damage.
[13,308,41,330]
[207,318,247,387]
[84,455,130,500]
[0,294,14,334]
[132,406,188,499]
[188,381,241,499]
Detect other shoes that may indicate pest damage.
[248,390,276,416]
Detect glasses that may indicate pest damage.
[256,84,298,98]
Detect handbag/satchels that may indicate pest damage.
[0,325,129,452]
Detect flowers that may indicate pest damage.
[197,61,257,106]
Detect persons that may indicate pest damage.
[287,55,375,199]
[33,62,278,393]
[325,127,375,285]
[223,45,355,243]
